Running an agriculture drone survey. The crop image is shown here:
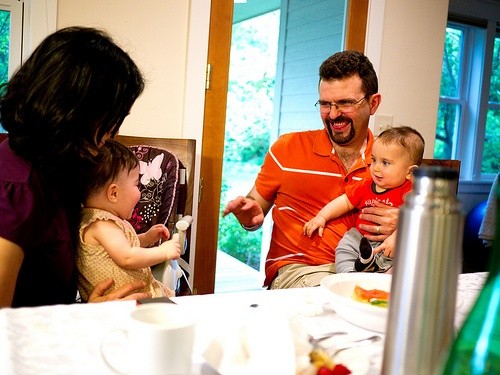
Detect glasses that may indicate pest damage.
[313,95,368,114]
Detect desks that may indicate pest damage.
[0,272,493,375]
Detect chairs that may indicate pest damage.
[123,144,193,298]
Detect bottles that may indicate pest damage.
[444,230,500,375]
[378,166,464,374]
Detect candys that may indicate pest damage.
[354,286,390,308]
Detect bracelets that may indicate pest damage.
[241,196,264,232]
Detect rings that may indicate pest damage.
[377,226,380,234]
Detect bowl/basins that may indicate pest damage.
[320,272,393,336]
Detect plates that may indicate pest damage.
[101,322,228,374]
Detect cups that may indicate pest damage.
[129,302,195,374]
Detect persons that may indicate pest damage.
[220,50,426,289]
[0,26,182,310]
